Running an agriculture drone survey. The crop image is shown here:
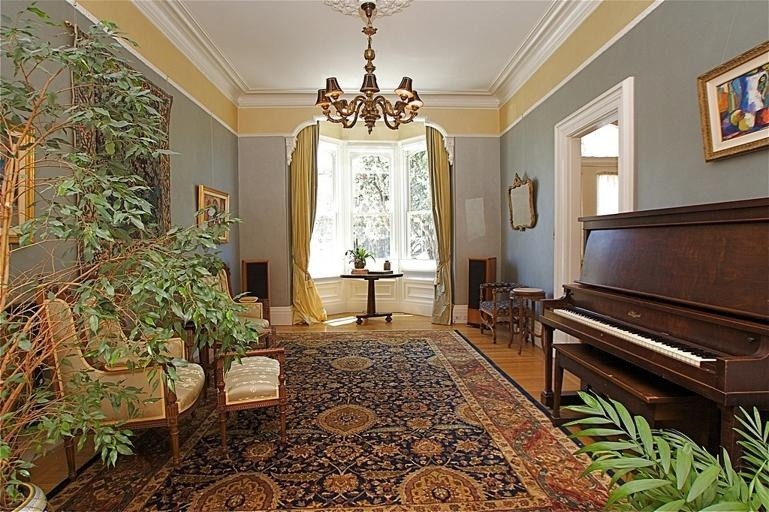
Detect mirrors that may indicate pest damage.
[508,173,537,232]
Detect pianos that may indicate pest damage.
[538,199,768,492]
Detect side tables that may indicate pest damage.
[508,288,547,355]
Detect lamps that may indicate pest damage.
[315,0,424,135]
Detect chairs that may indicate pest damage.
[199,263,271,346]
[36,284,206,482]
[480,282,529,344]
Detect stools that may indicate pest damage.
[216,347,287,452]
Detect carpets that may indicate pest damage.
[45,330,645,512]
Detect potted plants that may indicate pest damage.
[0,0,259,512]
[345,237,376,269]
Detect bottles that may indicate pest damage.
[383,261,391,270]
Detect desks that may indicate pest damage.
[340,273,403,325]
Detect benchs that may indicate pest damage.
[552,344,711,475]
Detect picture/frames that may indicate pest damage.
[198,184,231,244]
[697,41,769,163]
[0,117,35,244]
